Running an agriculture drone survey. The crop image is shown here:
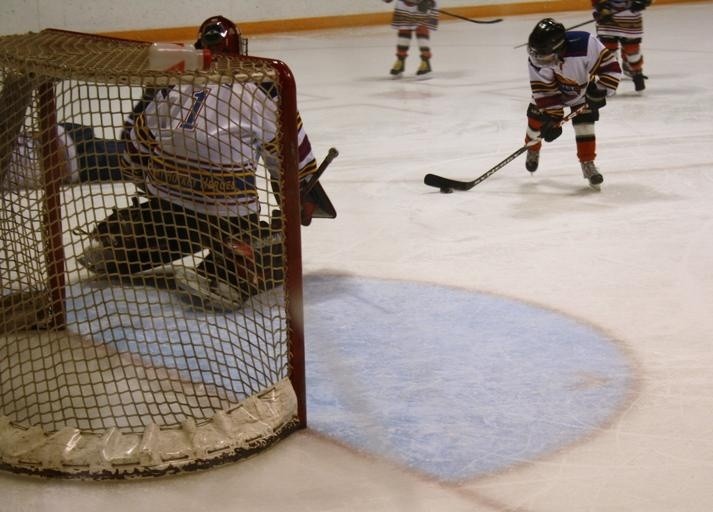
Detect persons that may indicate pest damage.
[591,1,652,95]
[68,12,341,314]
[0,122,145,192]
[522,17,619,191]
[383,0,442,81]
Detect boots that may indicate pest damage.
[389,58,404,74]
[416,58,431,75]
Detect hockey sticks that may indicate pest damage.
[513,8,629,64]
[402,0,504,24]
[424,103,593,192]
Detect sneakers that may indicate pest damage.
[580,163,603,184]
[525,151,539,172]
[632,74,645,91]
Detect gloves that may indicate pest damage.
[583,82,607,109]
[629,1,647,13]
[417,1,432,14]
[540,122,562,142]
[592,7,611,24]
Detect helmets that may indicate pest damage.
[195,16,249,59]
[526,18,567,68]
